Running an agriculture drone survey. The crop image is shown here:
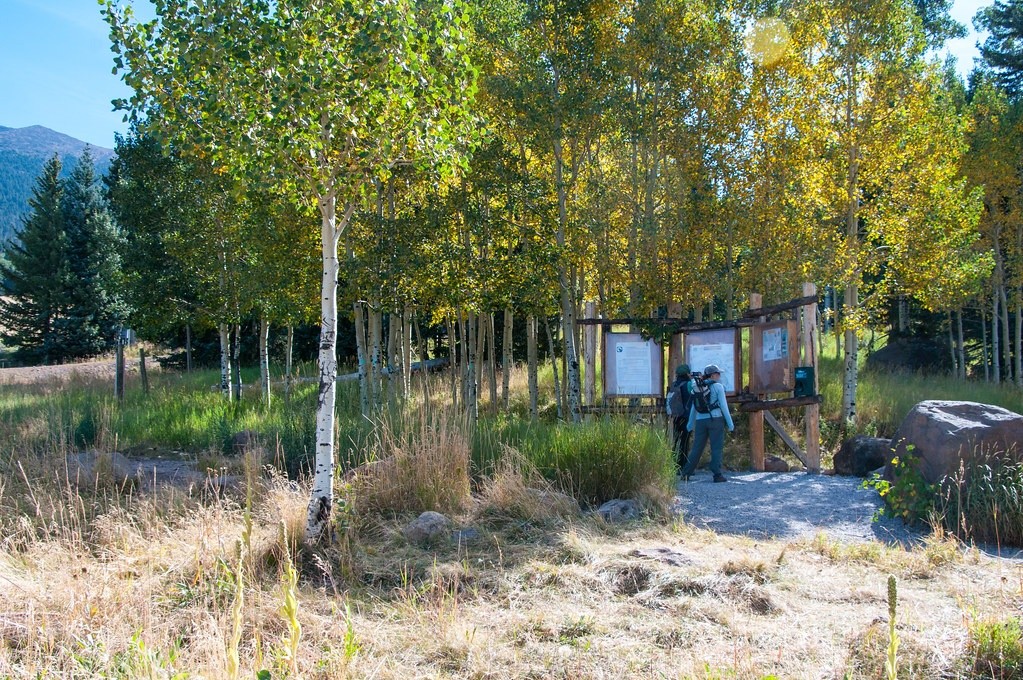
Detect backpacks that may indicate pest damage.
[670,380,690,416]
[693,382,721,422]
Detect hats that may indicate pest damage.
[675,364,692,376]
[704,364,724,376]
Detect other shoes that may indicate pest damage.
[680,475,689,483]
[689,471,696,476]
[714,475,728,483]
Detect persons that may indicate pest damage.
[681,365,734,483]
[666,364,702,477]
